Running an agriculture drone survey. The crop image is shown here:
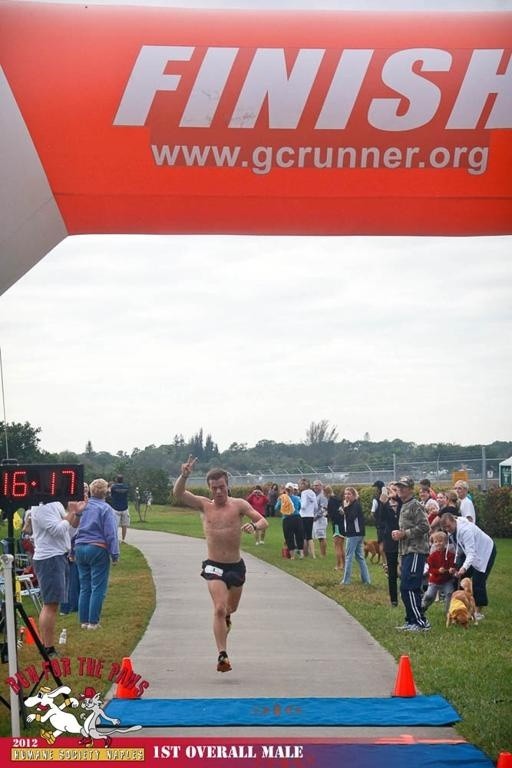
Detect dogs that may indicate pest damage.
[362,538,382,564]
[444,576,479,631]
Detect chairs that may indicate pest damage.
[15,573,42,615]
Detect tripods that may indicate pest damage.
[0,515,73,728]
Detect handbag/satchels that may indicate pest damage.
[282,541,290,559]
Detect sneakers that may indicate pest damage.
[80,623,88,629]
[217,651,232,671]
[86,623,101,630]
[395,623,431,632]
[225,617,231,636]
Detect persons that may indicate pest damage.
[370,477,388,569]
[299,478,317,559]
[59,549,79,617]
[28,496,90,661]
[312,479,329,558]
[293,483,300,498]
[265,483,278,517]
[419,476,437,501]
[274,482,304,560]
[455,480,475,526]
[422,532,459,617]
[439,512,496,627]
[391,475,434,633]
[171,451,269,674]
[375,481,403,607]
[74,478,122,631]
[246,485,269,545]
[22,511,37,579]
[435,492,447,512]
[438,489,460,520]
[338,485,371,587]
[320,484,345,570]
[83,483,91,496]
[110,473,130,542]
[417,486,439,513]
[425,502,441,525]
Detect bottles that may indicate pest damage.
[59,628,67,644]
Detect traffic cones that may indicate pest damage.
[496,751,511,768]
[113,657,142,697]
[389,655,420,696]
[22,616,42,643]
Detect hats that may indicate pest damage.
[372,481,385,487]
[254,485,261,490]
[393,476,415,487]
[285,482,294,489]
[294,485,299,490]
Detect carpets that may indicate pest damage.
[93,694,462,726]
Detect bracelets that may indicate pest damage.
[75,512,84,518]
[250,522,258,531]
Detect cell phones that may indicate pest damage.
[381,487,388,496]
